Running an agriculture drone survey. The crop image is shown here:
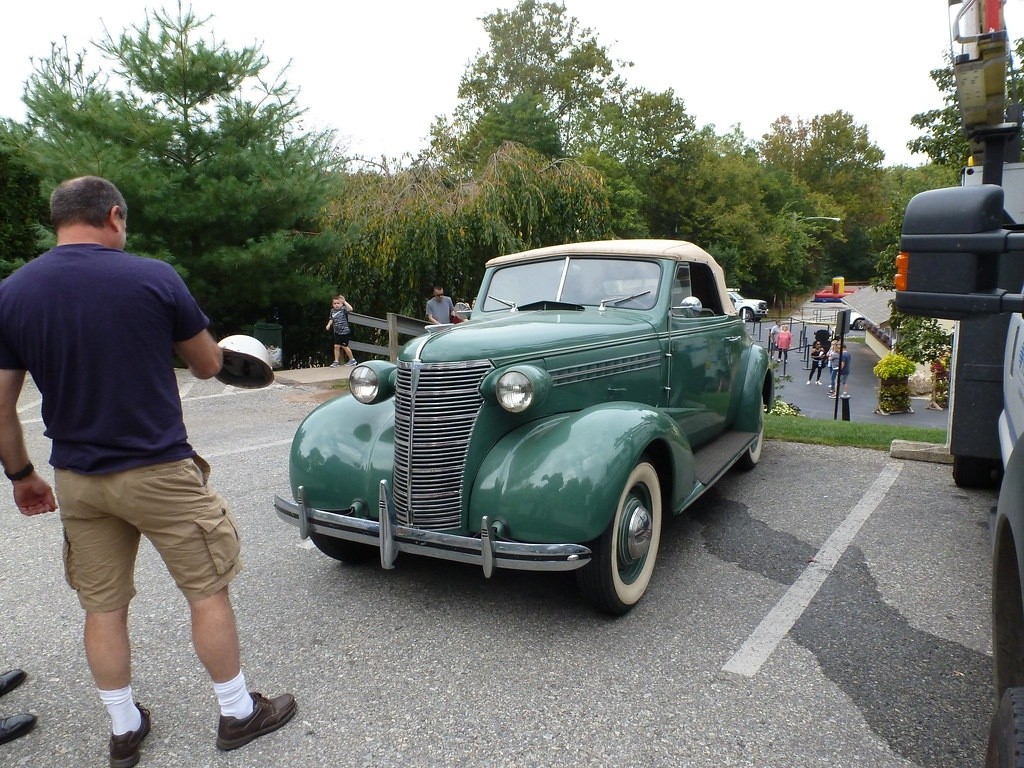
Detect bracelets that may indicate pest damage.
[4,462,34,481]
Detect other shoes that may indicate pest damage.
[806,380,812,385]
[778,358,781,364]
[785,360,788,365]
[770,355,774,359]
[827,390,835,395]
[838,395,842,399]
[815,381,823,385]
[828,394,837,399]
[827,385,832,388]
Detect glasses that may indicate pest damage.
[435,293,444,296]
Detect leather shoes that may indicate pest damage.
[216,692,298,751]
[0,669,27,698]
[109,703,151,768]
[0,713,38,746]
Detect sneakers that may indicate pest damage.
[344,359,358,367]
[330,360,340,367]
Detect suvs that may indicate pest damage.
[725,288,769,323]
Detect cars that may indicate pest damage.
[269,235,775,611]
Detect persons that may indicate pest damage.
[326,295,358,368]
[770,320,851,398]
[1,175,297,768]
[425,284,477,324]
